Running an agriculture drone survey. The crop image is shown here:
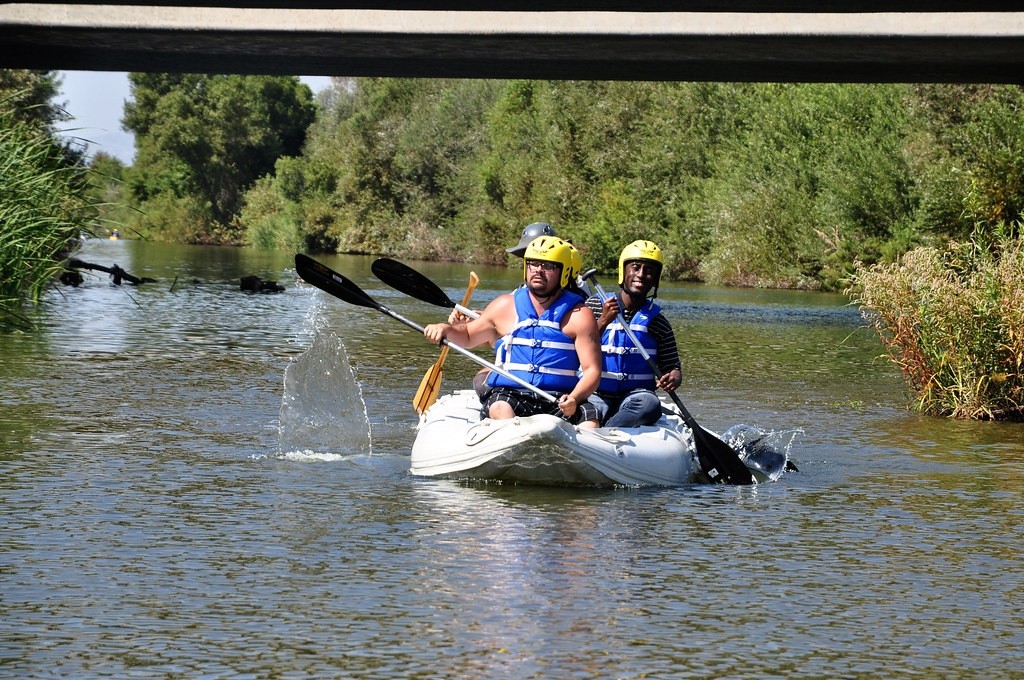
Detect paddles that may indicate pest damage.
[412,269,480,417]
[293,252,562,406]
[581,267,757,487]
[370,256,481,320]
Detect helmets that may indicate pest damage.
[523,235,572,287]
[564,240,582,279]
[505,222,555,259]
[618,240,663,285]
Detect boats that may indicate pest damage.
[409,387,696,487]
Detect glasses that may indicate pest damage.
[525,260,562,272]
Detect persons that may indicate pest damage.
[442,220,560,325]
[421,234,603,431]
[583,237,683,430]
